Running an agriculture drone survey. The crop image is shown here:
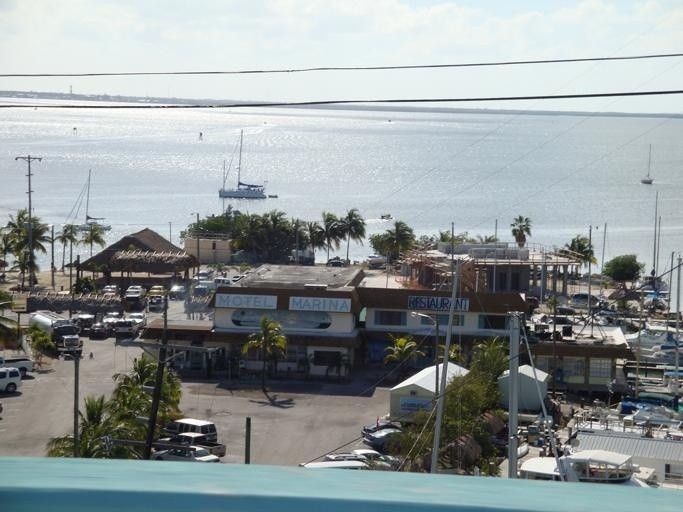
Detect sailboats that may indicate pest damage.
[547,190,683,428]
[518,318,659,487]
[60,169,112,231]
[219,128,267,198]
[640,143,656,184]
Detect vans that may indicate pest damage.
[0,367,24,394]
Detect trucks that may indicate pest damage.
[28,310,84,359]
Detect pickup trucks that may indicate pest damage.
[0,357,33,379]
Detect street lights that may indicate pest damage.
[411,311,439,474]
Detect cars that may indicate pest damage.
[298,422,409,471]
[68,270,253,340]
[150,418,226,462]
